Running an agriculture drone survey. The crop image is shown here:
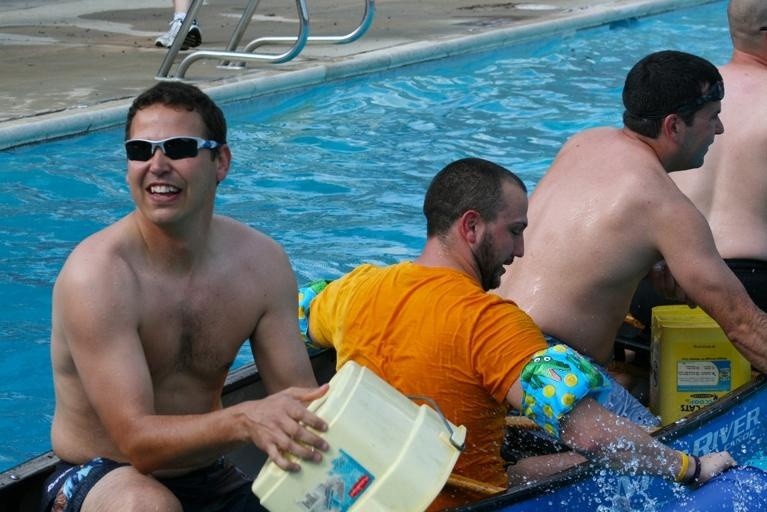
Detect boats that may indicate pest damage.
[1,344,763,512]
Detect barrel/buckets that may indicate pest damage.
[252,361,466,512]
[651,305,752,426]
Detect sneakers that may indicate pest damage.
[156,14,202,51]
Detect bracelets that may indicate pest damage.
[674,447,689,483]
[688,454,702,486]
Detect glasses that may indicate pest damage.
[644,81,725,117]
[123,136,219,160]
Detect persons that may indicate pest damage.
[623,0,767,327]
[39,77,330,512]
[155,1,202,50]
[478,48,766,437]
[295,155,737,511]
